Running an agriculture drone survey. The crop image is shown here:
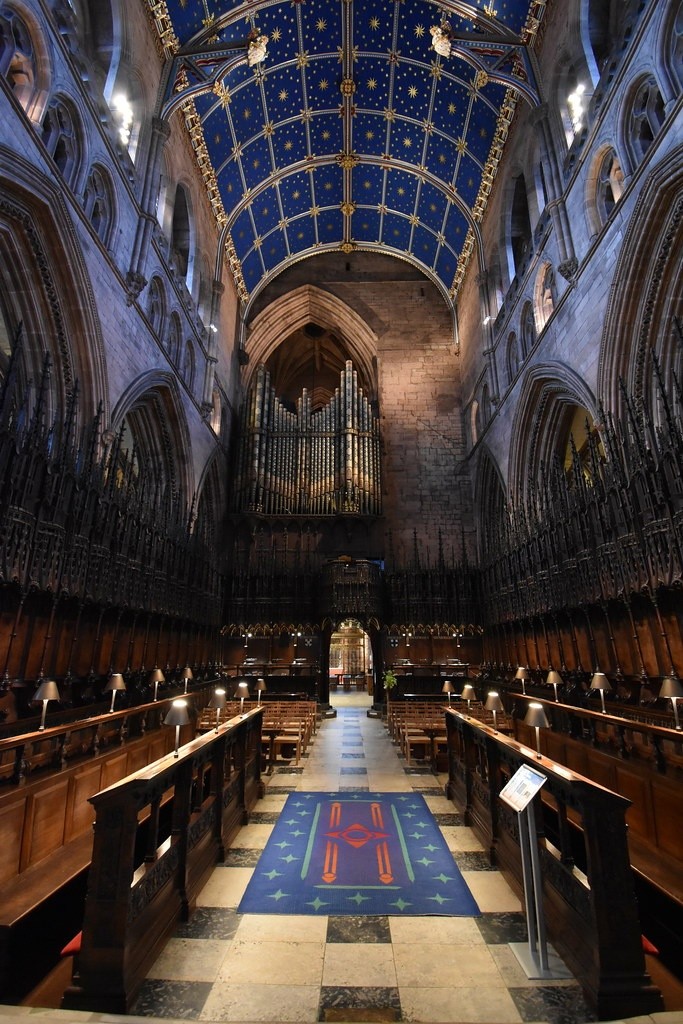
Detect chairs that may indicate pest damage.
[197,700,321,766]
[388,700,513,766]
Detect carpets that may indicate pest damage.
[236,791,483,917]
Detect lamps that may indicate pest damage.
[208,689,226,734]
[254,679,267,708]
[546,671,564,703]
[659,678,683,732]
[484,692,505,735]
[442,681,455,709]
[149,669,165,702]
[163,700,189,759]
[180,667,193,695]
[590,673,612,714]
[204,323,217,333]
[106,673,126,713]
[460,685,477,719]
[233,683,250,719]
[515,667,529,696]
[32,681,60,732]
[482,315,496,325]
[524,704,550,759]
